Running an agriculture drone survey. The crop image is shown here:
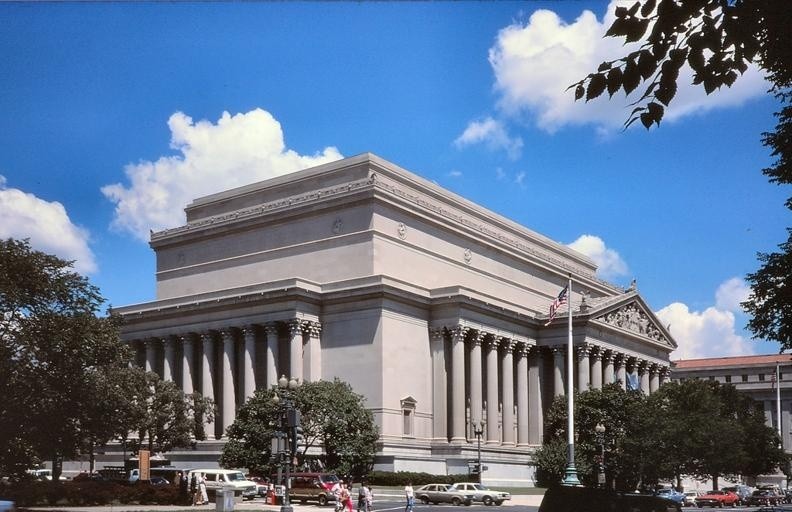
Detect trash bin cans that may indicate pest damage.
[216,489,235,512]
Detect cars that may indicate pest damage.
[414,483,512,506]
[25,469,68,482]
[655,486,792,507]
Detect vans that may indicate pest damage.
[270,472,340,506]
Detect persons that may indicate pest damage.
[331,474,375,512]
[405,480,414,512]
[173,471,209,505]
[83,470,89,478]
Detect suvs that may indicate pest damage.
[74,459,273,500]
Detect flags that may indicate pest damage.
[627,372,642,395]
[545,285,569,329]
[770,367,777,393]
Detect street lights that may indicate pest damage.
[273,373,298,489]
[470,419,487,484]
[595,422,607,488]
